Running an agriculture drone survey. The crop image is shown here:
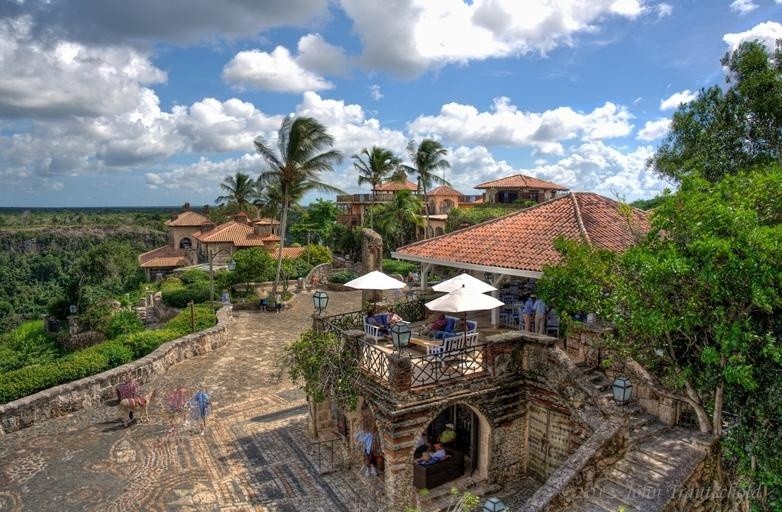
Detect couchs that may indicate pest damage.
[413,448,465,491]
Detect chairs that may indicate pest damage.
[499,276,565,339]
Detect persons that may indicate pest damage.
[259,287,268,313]
[387,308,403,326]
[452,312,468,335]
[423,313,448,336]
[523,293,537,332]
[533,296,549,334]
[416,433,446,467]
[367,309,393,344]
[354,428,379,477]
[438,423,457,444]
[275,291,282,314]
[191,383,211,435]
[172,383,188,412]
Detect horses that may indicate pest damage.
[119,388,157,427]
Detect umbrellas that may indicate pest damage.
[431,273,499,294]
[425,285,506,347]
[343,269,408,313]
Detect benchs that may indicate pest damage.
[363,310,408,346]
[421,316,478,346]
[424,334,477,364]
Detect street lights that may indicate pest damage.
[307,230,323,264]
[209,248,237,312]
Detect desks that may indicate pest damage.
[410,336,443,358]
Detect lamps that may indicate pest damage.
[481,494,506,511]
[391,321,410,351]
[612,375,632,405]
[312,290,328,311]
